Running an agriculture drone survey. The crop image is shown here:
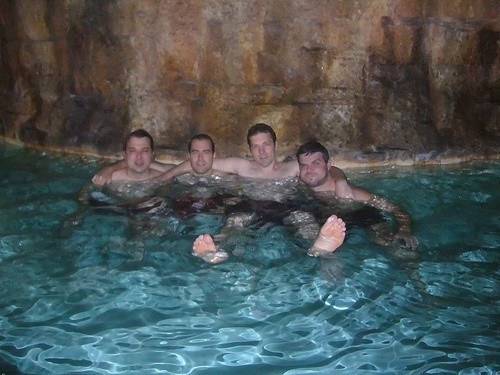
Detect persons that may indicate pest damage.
[93,130,418,250]
[157,123,356,263]
